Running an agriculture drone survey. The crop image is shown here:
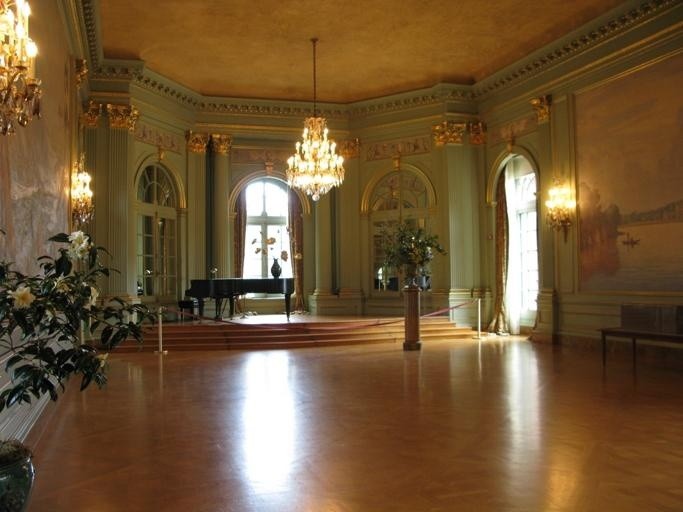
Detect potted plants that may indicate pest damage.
[250,230,289,277]
[378,221,449,289]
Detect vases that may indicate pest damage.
[0,436,34,512]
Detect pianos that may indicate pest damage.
[185,277,294,317]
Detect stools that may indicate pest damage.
[179,300,195,322]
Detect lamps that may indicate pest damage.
[545,176,577,234]
[71,153,95,224]
[285,38,345,202]
[2,0,44,137]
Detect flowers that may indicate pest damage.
[0,226,158,411]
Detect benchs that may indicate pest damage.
[597,302,683,377]
